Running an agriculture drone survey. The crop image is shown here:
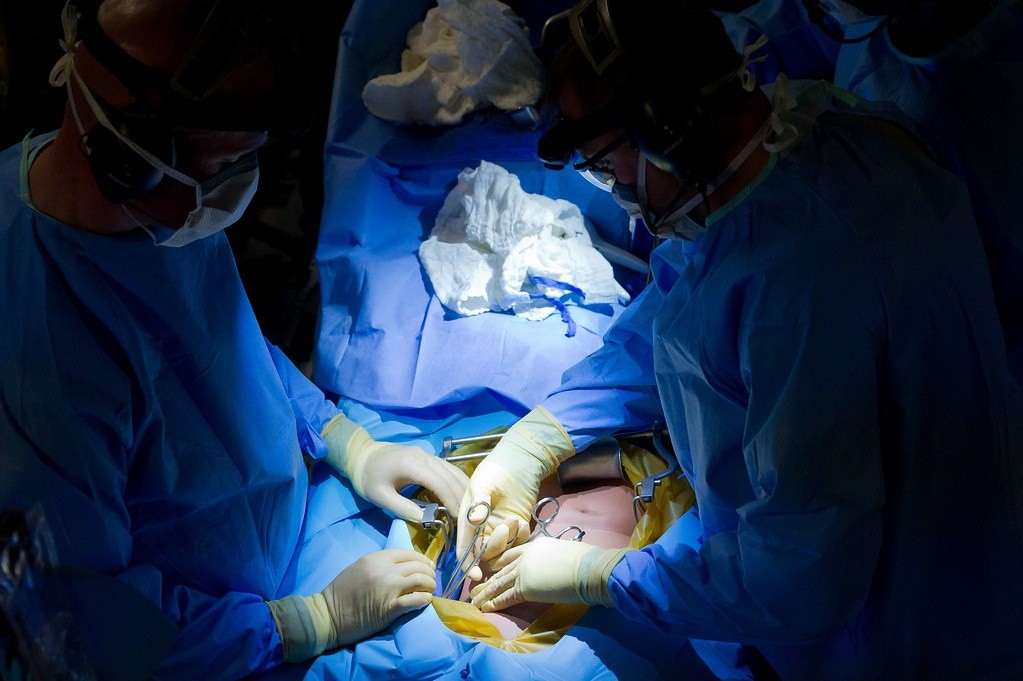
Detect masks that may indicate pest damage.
[611,104,686,244]
[86,52,259,248]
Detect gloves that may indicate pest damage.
[470,537,637,613]
[456,405,576,581]
[320,413,470,524]
[264,549,437,662]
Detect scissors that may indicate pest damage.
[524,496,586,543]
[441,501,517,603]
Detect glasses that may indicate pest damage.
[573,133,626,192]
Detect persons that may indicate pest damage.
[0,0,470,681]
[455,0,1022,681]
